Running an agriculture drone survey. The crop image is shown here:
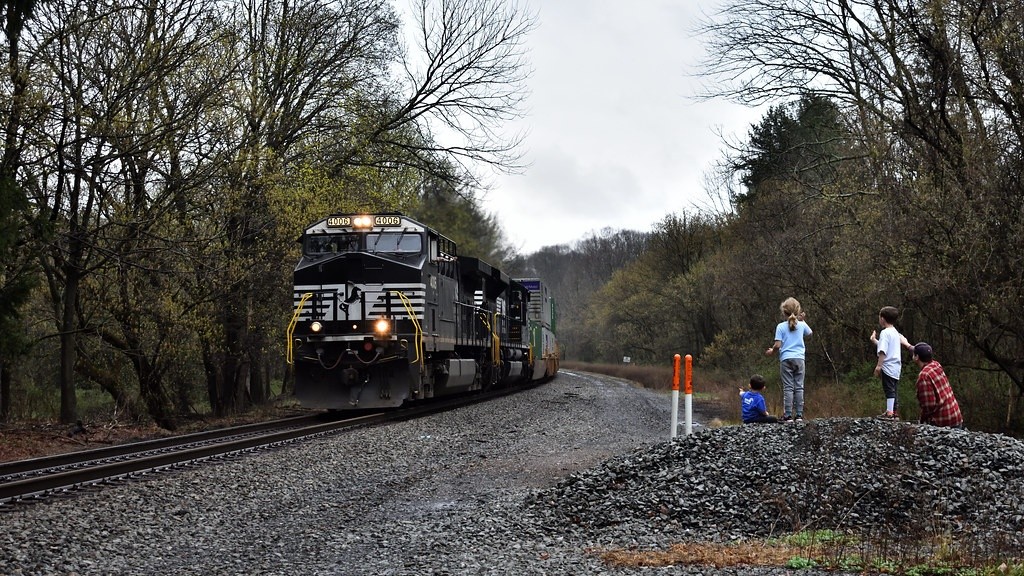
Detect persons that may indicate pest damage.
[739,374,778,424]
[898,333,963,429]
[870,306,902,422]
[766,297,813,423]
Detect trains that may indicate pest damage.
[276,210,560,413]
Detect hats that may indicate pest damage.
[907,342,932,359]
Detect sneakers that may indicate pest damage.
[795,413,803,423]
[876,410,895,421]
[776,412,793,423]
[892,413,899,422]
